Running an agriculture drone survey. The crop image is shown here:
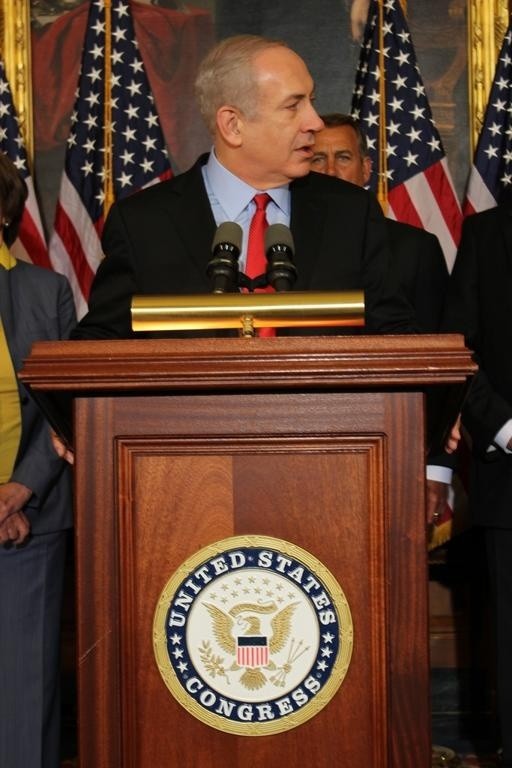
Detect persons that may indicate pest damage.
[303,110,455,461]
[41,29,472,533]
[0,144,85,767]
[426,186,512,768]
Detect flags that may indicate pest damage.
[348,0,472,279]
[45,0,180,324]
[0,56,53,274]
[460,13,512,232]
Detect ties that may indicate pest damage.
[237,192,278,339]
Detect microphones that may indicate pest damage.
[264,223,298,294]
[204,221,243,294]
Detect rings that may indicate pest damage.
[434,511,441,521]
